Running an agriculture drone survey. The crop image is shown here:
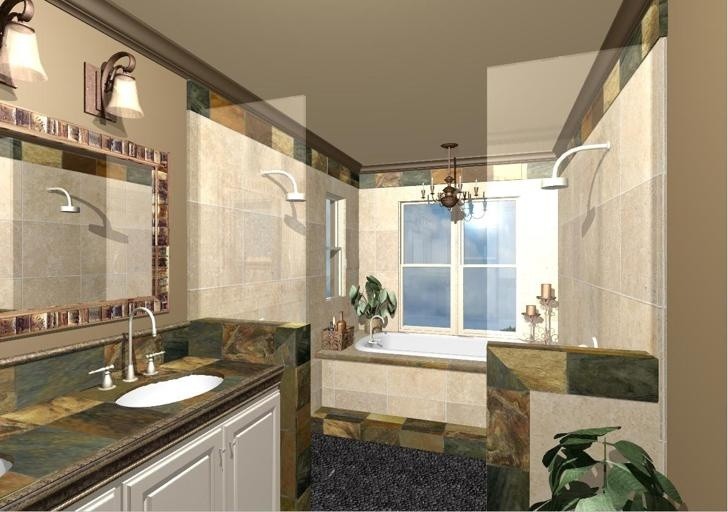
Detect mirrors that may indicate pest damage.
[1,102,170,342]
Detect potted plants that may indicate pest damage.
[349,275,397,333]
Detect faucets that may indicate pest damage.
[121,307,157,382]
[370,314,385,341]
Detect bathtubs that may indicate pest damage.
[355,332,486,364]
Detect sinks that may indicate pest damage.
[116,371,225,407]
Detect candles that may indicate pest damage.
[542,283,551,299]
[526,304,536,315]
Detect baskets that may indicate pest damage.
[321,326,354,351]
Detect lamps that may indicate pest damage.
[84,52,144,122]
[0,1,47,90]
[420,142,479,208]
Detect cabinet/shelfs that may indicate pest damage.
[47,382,281,512]
